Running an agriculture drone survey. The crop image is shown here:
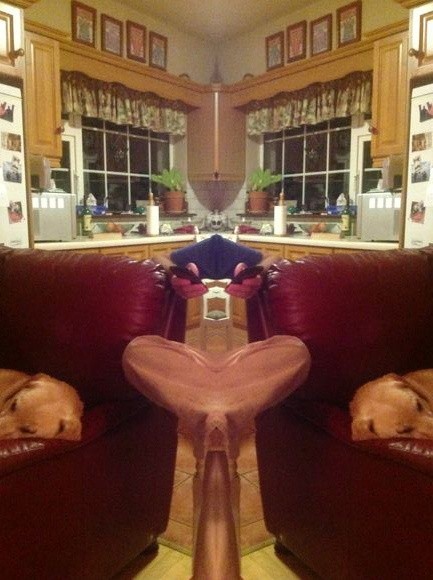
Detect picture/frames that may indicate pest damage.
[70,1,97,48]
[264,30,284,70]
[126,19,147,64]
[99,13,123,57]
[285,19,307,63]
[335,1,362,49]
[147,31,168,71]
[308,13,332,59]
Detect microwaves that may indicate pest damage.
[356,192,401,242]
[30,191,76,242]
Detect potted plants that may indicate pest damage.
[246,165,283,211]
[150,167,186,212]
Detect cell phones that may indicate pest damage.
[225,266,263,289]
[170,266,207,294]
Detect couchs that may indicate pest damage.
[248,247,433,578]
[0,240,188,579]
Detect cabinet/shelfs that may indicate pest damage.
[367,30,409,168]
[408,0,432,84]
[0,1,25,85]
[150,242,200,330]
[285,244,334,258]
[233,240,283,327]
[22,31,64,169]
[100,246,148,262]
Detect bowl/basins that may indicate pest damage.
[297,223,336,234]
[96,223,134,235]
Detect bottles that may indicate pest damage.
[80,194,92,236]
[340,195,351,238]
